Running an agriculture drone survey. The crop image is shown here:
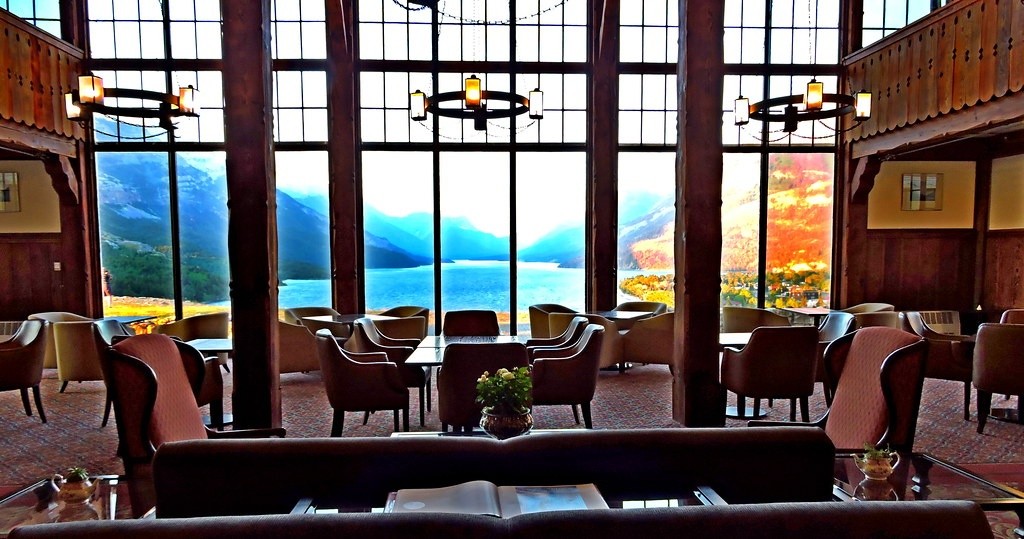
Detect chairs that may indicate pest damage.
[898,311,976,420]
[972,323,1024,434]
[28,312,94,369]
[748,326,927,458]
[443,310,500,336]
[108,333,286,519]
[529,303,580,338]
[610,301,667,316]
[354,317,431,426]
[723,305,791,333]
[93,319,182,388]
[526,316,589,364]
[53,321,104,392]
[379,306,430,337]
[769,311,856,407]
[110,334,224,430]
[152,311,230,373]
[623,308,674,374]
[285,307,350,348]
[853,311,896,329]
[436,343,533,432]
[840,303,895,313]
[721,326,819,423]
[278,317,320,373]
[532,324,605,429]
[315,328,410,437]
[0,318,47,423]
[549,312,623,373]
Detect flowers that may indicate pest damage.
[474,365,532,412]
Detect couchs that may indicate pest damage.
[4,426,997,539]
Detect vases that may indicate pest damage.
[477,406,534,440]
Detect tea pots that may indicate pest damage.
[51,474,103,503]
[850,452,900,480]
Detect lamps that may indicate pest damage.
[732,0,872,142]
[64,0,202,140]
[409,0,544,142]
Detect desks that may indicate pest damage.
[719,332,767,419]
[91,315,157,324]
[1,449,1024,539]
[302,314,401,337]
[417,335,518,347]
[777,307,837,327]
[182,338,233,427]
[595,311,653,371]
[405,348,445,365]
